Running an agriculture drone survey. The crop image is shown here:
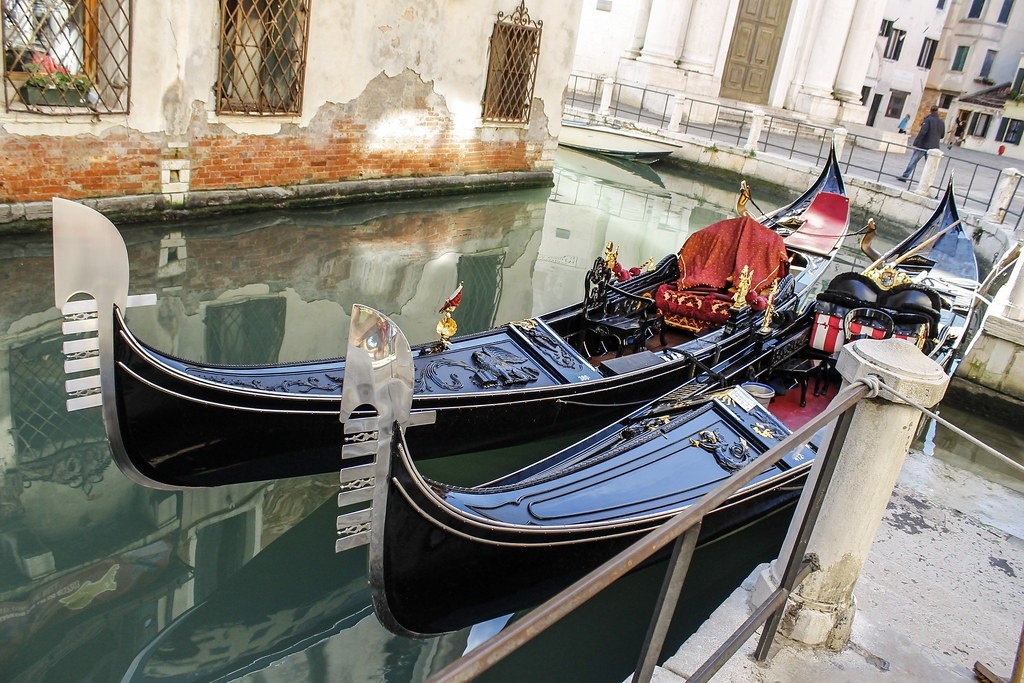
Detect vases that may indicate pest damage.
[18,84,89,107]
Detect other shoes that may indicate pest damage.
[896,176,906,182]
[947,146,952,150]
[962,139,966,143]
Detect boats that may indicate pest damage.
[51,136,853,493]
[338,169,980,641]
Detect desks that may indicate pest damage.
[765,359,823,407]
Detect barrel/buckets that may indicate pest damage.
[742,382,775,409]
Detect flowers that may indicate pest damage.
[20,51,91,91]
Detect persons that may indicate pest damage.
[947,117,966,150]
[897,114,910,135]
[896,105,945,183]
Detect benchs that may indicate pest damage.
[654,217,789,346]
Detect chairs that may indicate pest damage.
[577,257,669,358]
[821,306,895,395]
